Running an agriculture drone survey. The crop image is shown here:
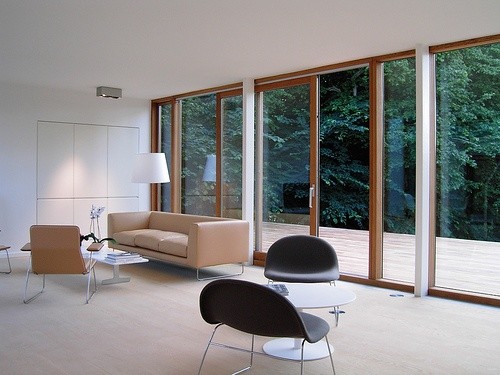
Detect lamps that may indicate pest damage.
[132,153,170,184]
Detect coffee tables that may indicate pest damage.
[260,283,357,361]
[80,242,149,285]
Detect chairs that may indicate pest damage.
[21,224,104,304]
[264,235,345,326]
[198,279,335,375]
[0,230,11,274]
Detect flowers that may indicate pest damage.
[90,204,104,220]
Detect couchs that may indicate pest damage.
[107,211,249,280]
